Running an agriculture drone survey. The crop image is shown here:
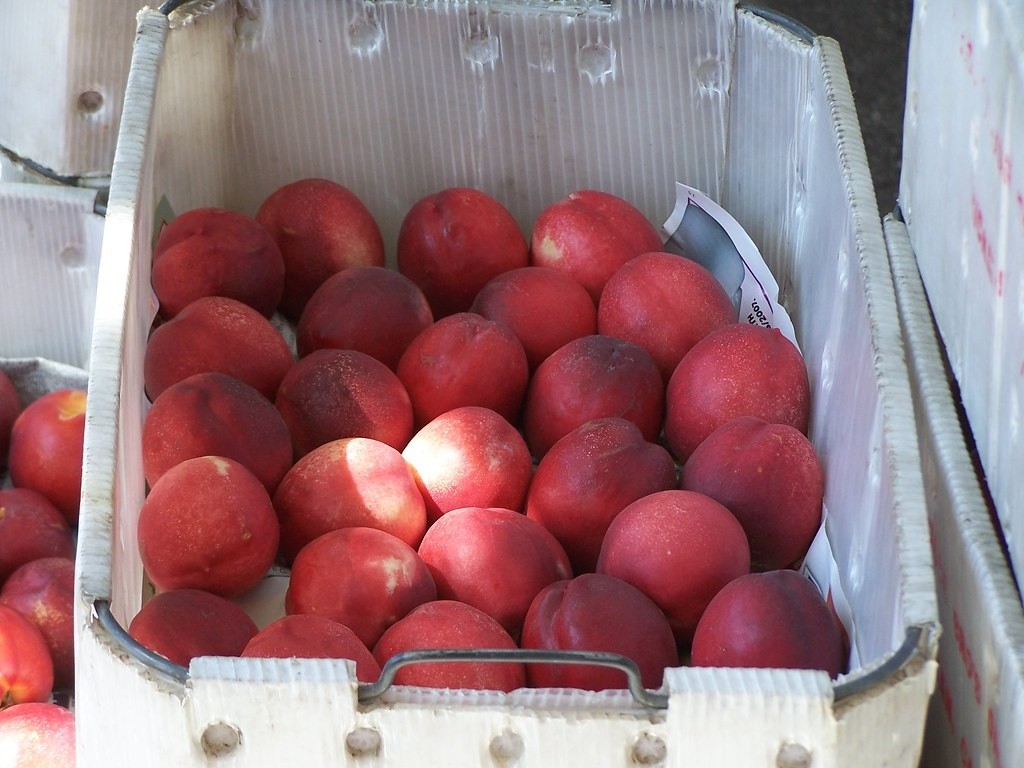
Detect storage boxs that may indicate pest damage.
[0,0,1024,768]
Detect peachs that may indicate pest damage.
[124,179,843,690]
[0,367,88,768]
